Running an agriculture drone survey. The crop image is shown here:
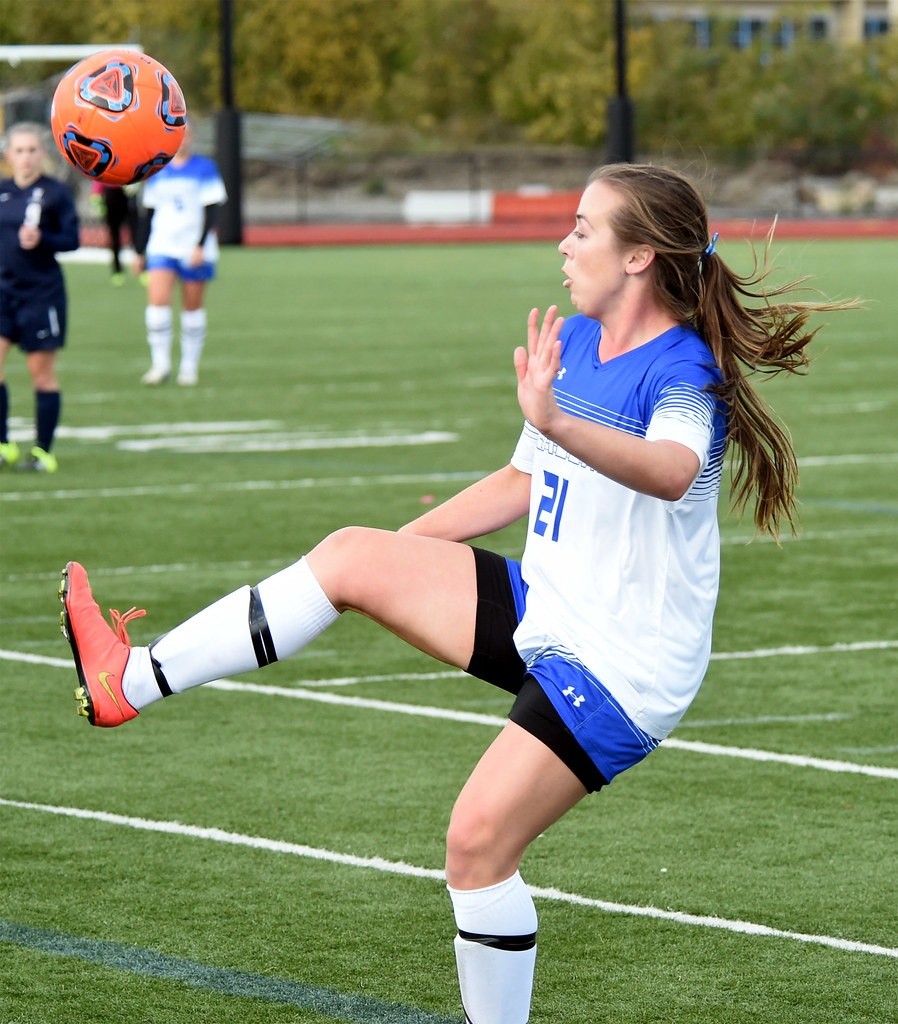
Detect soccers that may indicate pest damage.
[51,49,188,187]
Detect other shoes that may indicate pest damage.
[27,447,57,473]
[139,367,170,384]
[138,270,149,288]
[176,366,198,385]
[111,267,125,286]
[1,441,21,471]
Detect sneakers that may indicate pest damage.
[59,561,147,727]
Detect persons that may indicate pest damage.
[58,162,870,1024]
[88,178,153,291]
[0,123,80,473]
[137,113,229,388]
